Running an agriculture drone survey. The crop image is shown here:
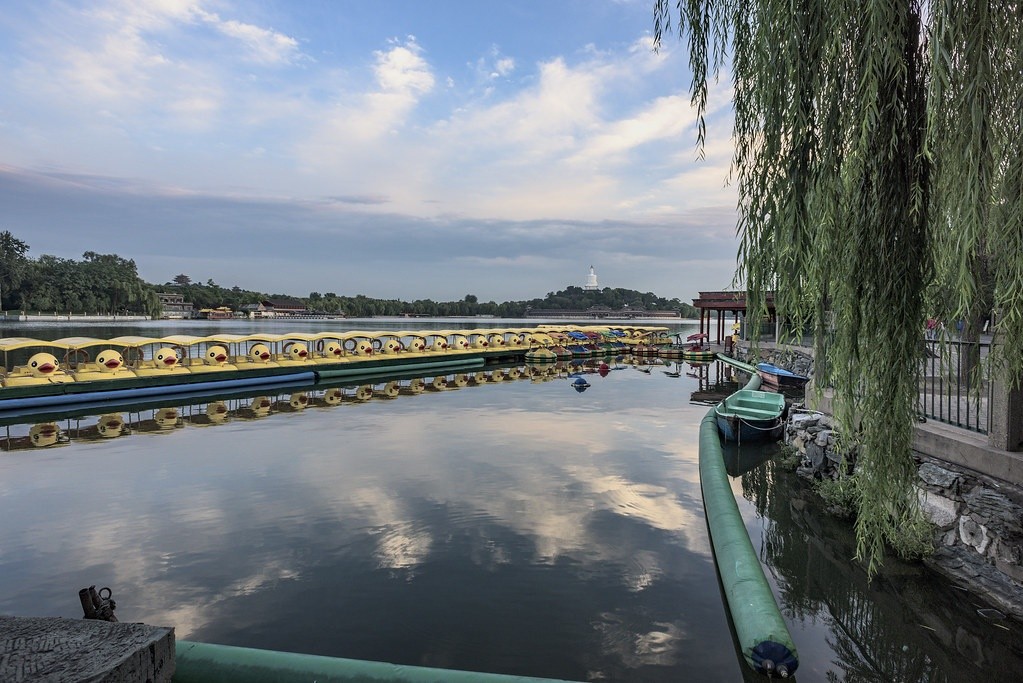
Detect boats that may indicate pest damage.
[0,324,716,451]
[197,307,247,320]
[753,360,811,392]
[714,389,787,445]
